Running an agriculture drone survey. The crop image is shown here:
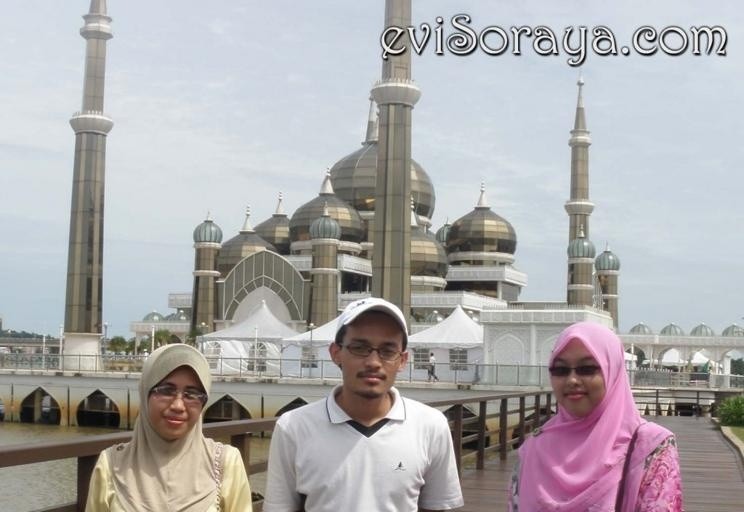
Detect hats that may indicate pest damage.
[333,297,409,352]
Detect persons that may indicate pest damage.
[508,320,682,511]
[262,298,466,511]
[426,353,439,382]
[85,342,253,511]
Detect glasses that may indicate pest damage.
[338,342,406,361]
[549,365,601,376]
[150,385,208,403]
